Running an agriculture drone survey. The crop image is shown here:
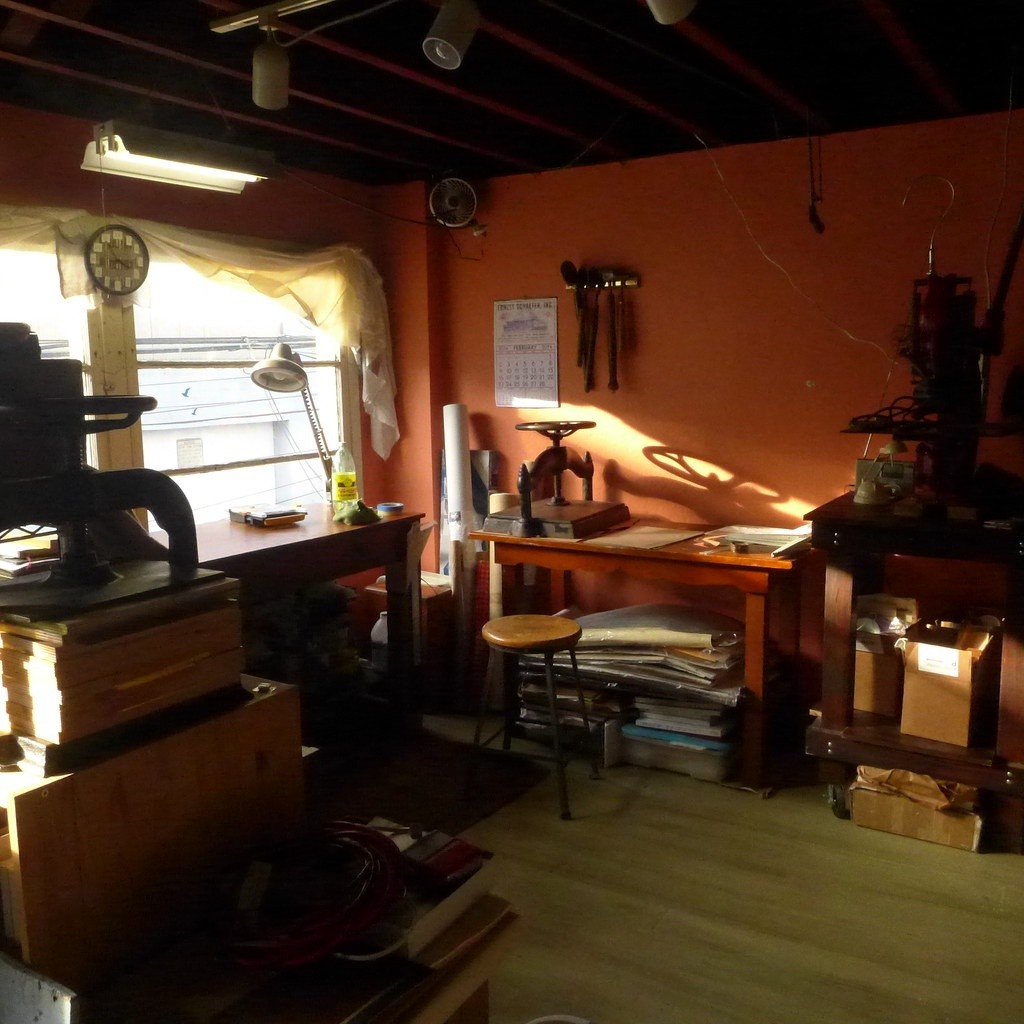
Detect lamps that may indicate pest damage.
[82,118,270,197]
[646,0,696,25]
[421,0,480,70]
[252,14,290,110]
[250,344,334,508]
[853,441,910,504]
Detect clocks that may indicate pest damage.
[83,224,150,295]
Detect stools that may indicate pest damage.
[362,584,451,658]
[464,615,600,822]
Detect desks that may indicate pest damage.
[145,502,427,762]
[802,488,1024,850]
[467,522,828,790]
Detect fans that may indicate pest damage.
[428,178,477,229]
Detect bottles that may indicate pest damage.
[371,611,387,668]
[332,442,359,515]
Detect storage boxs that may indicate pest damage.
[850,782,984,851]
[897,606,1003,749]
[852,593,920,721]
